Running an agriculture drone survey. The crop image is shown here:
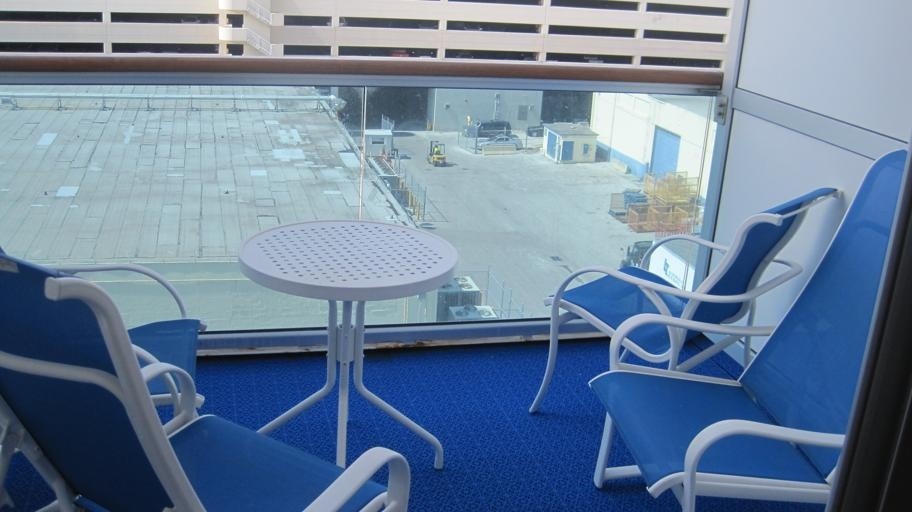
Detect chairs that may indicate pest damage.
[528,186,834,415]
[0,245,207,419]
[586,150,911,512]
[0,255,411,512]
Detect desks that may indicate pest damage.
[240,221,459,471]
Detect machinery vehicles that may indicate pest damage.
[427,141,446,167]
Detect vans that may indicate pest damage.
[464,120,511,137]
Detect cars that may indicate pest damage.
[477,135,522,150]
[527,125,544,136]
